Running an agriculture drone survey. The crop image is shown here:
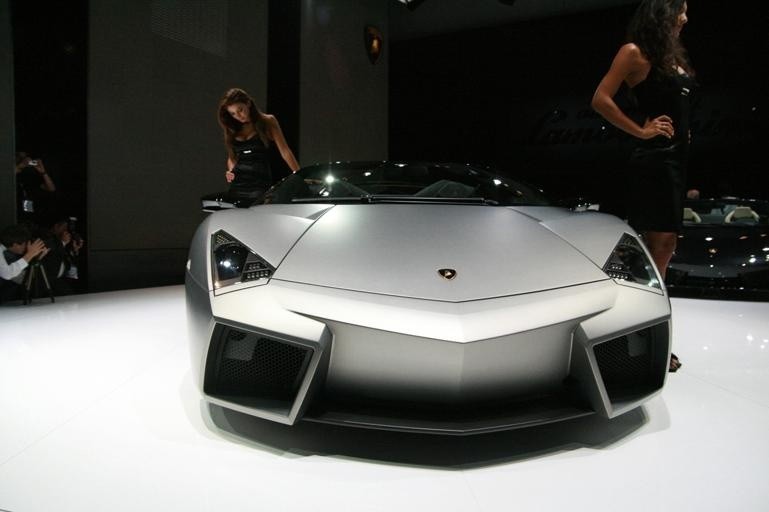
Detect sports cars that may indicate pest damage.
[662,194,768,301]
[184,156,673,437]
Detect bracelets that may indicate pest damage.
[41,171,47,175]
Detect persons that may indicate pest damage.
[591,0,695,371]
[218,89,324,199]
[15,148,56,223]
[36,216,83,298]
[0,223,46,307]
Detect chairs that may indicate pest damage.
[682,206,760,224]
[304,179,476,199]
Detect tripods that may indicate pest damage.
[22,260,55,305]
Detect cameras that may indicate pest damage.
[67,216,80,242]
[28,158,39,166]
